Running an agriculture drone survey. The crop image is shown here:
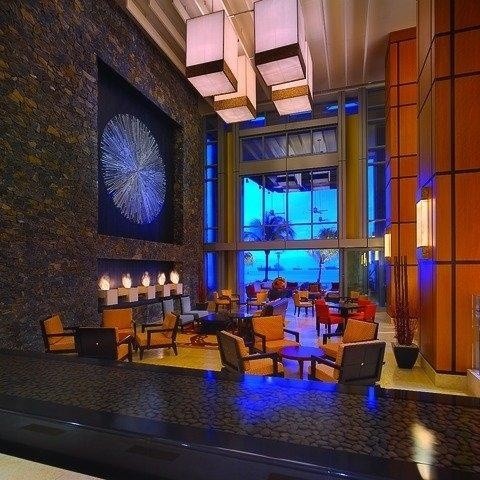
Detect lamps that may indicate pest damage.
[276,172,331,193]
[185,0,315,124]
[414,187,434,261]
[383,227,392,264]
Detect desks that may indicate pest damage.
[278,346,326,380]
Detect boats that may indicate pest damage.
[309,265,339,271]
[257,264,286,272]
[293,265,303,270]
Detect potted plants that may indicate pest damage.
[390,306,419,369]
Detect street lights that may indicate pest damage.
[275,252,283,278]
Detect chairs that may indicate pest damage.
[79,326,132,362]
[41,315,78,353]
[249,314,299,367]
[133,311,179,361]
[318,319,379,365]
[308,339,385,387]
[102,308,137,354]
[216,330,284,378]
[99,283,377,337]
